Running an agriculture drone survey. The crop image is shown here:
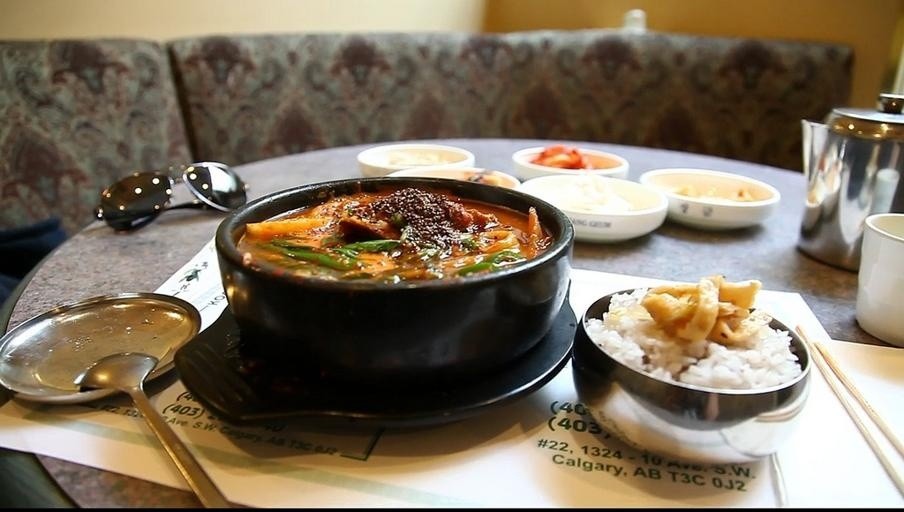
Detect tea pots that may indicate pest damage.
[794,92,904,272]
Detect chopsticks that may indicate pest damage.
[792,322,904,502]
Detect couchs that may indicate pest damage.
[165,29,851,175]
[0,41,197,250]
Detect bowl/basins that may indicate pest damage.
[512,146,631,182]
[569,286,812,468]
[521,174,669,244]
[355,142,475,179]
[385,165,521,193]
[640,168,781,232]
[214,177,575,413]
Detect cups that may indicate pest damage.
[856,214,904,347]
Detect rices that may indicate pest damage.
[588,289,802,390]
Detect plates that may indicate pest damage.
[172,274,577,434]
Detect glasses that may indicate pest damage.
[94,162,247,231]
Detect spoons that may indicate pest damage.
[72,351,233,509]
